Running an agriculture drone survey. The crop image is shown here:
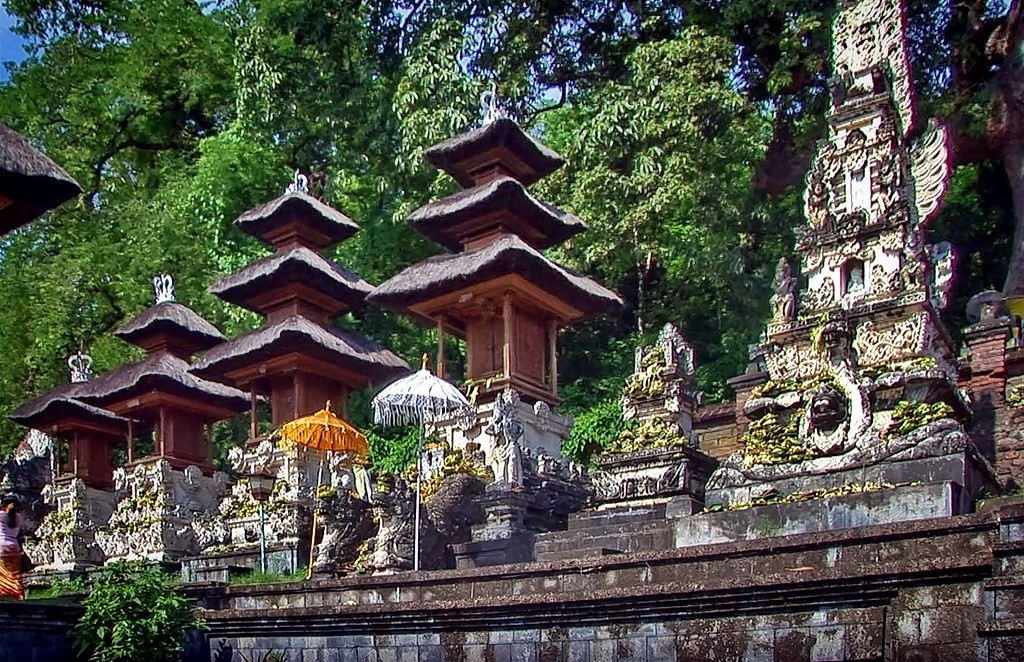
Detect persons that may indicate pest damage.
[810,182,830,232]
[1,493,26,601]
[900,229,927,291]
[768,258,798,324]
[486,398,526,486]
[872,158,897,216]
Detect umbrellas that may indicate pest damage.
[277,399,369,581]
[369,351,472,570]
